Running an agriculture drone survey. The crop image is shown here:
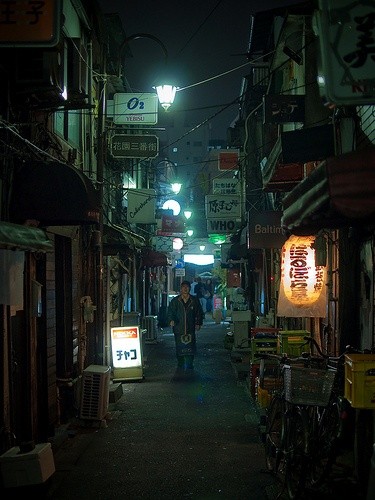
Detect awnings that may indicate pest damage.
[9,160,172,268]
[278,149,375,236]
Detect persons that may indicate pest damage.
[167,277,215,381]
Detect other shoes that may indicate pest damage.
[187,365,193,369]
[177,361,184,366]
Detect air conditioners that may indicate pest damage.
[10,37,89,110]
[79,365,111,420]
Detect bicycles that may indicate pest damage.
[254,334,367,500]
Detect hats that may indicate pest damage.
[181,281,190,285]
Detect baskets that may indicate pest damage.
[345,353,375,409]
[281,364,337,406]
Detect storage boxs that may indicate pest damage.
[343,354,375,409]
[0,442,55,487]
[231,309,251,322]
[250,327,308,408]
